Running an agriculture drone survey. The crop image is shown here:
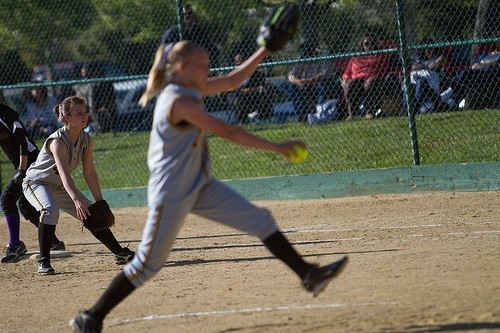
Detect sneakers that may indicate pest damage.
[51,241,66,250]
[1,241,27,263]
[302,256,348,296]
[69,309,102,333]
[37,257,55,274]
[114,247,135,265]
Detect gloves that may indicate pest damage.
[11,170,26,184]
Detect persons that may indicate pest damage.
[287,27,500,122]
[68,3,348,333]
[22,96,136,275]
[227,53,272,127]
[0,102,66,263]
[19,67,118,133]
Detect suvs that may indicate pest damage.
[30,58,156,125]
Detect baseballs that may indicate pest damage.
[288,145,309,163]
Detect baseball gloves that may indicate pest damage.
[83,200,115,232]
[256,1,301,52]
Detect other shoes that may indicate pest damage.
[421,103,434,112]
[345,114,355,121]
[365,112,373,119]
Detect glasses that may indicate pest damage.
[364,42,375,46]
